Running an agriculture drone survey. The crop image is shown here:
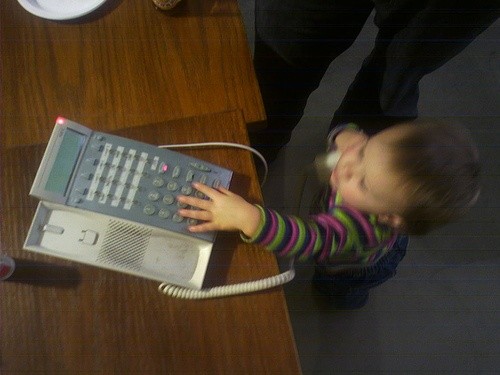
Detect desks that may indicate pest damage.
[1,1,268,146]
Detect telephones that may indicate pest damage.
[314,147,346,184]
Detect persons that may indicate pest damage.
[173,115,483,312]
[234,0,500,195]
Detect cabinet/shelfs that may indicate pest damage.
[0,106,305,375]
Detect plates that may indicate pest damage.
[17,0,107,20]
